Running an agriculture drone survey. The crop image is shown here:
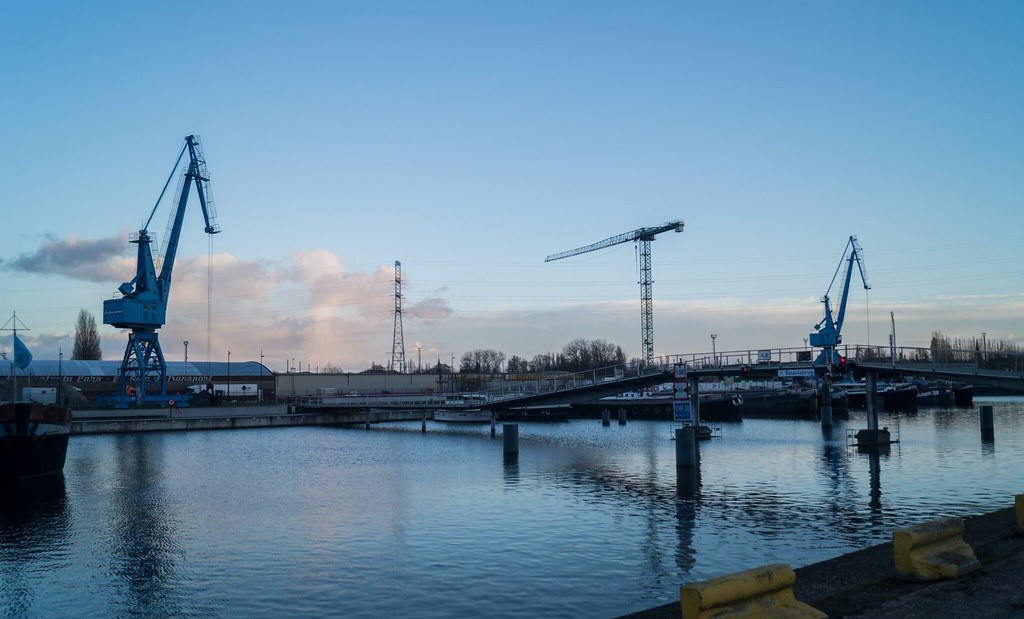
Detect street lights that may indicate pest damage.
[711,334,717,368]
[981,332,986,363]
[228,351,232,397]
[261,350,264,401]
[804,338,808,350]
[183,342,189,376]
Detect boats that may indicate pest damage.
[0,402,75,491]
[498,380,974,417]
[433,394,498,422]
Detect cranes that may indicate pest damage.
[810,233,874,366]
[543,220,686,370]
[101,133,220,407]
